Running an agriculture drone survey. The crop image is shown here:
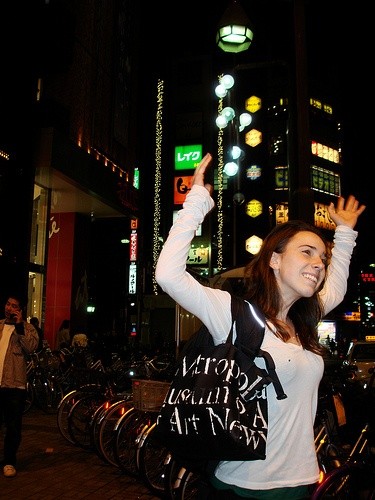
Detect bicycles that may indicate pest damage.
[22,338,375,500]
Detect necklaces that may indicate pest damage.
[291,335,297,339]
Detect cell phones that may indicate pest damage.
[10,310,20,319]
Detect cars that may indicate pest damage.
[345,336,375,388]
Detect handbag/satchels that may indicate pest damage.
[157,290,288,461]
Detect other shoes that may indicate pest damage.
[4,465,17,477]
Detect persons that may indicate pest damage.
[55,320,70,350]
[155,151,366,500]
[0,296,39,476]
